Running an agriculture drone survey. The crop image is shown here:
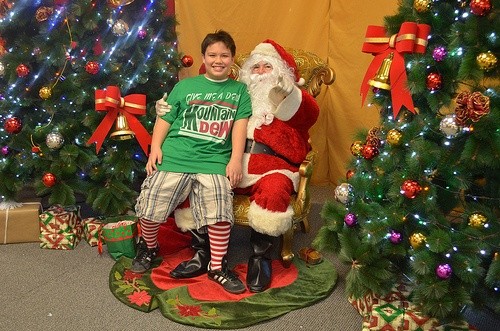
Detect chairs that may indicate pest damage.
[231,47,335,268]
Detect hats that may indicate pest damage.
[251,38,305,85]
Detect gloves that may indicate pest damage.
[274,74,293,96]
[156,93,172,117]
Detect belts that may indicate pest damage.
[244,139,279,155]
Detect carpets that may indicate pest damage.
[108,216,337,329]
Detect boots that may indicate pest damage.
[246,231,272,293]
[169,229,210,278]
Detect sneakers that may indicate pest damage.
[131,236,160,273]
[206,258,245,293]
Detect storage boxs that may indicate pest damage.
[0,198,105,250]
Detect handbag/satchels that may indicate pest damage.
[103,216,138,259]
